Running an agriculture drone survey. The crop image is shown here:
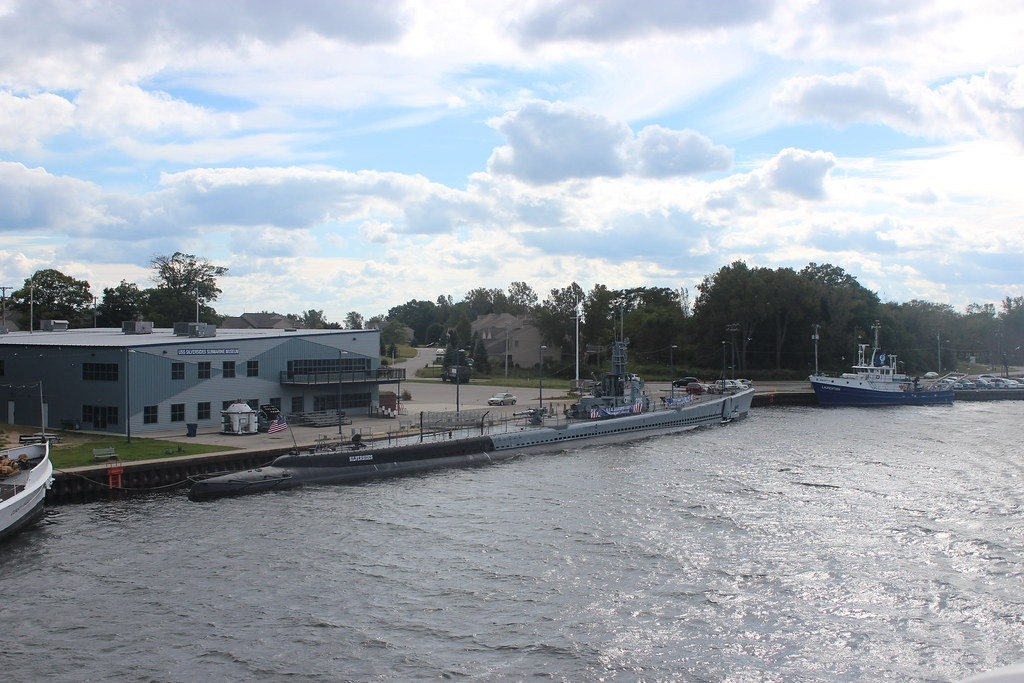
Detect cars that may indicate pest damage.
[952,373,1024,389]
[686,378,752,394]
[671,376,699,387]
[487,392,518,406]
[923,371,940,379]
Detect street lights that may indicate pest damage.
[539,345,546,409]
[127,349,135,445]
[670,343,679,398]
[339,350,349,433]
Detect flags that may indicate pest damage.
[268,415,287,434]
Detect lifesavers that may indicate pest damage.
[623,397,627,404]
[876,375,880,380]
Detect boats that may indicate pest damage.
[809,319,967,408]
[0,380,55,545]
[184,304,760,505]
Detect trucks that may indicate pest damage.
[440,364,470,383]
